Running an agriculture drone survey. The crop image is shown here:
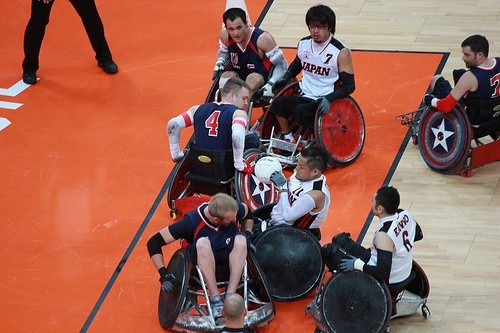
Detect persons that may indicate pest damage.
[211,8,288,103]
[256,144,331,235]
[146,194,254,320]
[323,187,423,301]
[22,0,117,84]
[219,294,254,333]
[423,35,500,133]
[168,78,266,173]
[273,5,355,155]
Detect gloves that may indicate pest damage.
[161,281,173,293]
[422,93,435,107]
[212,59,225,81]
[270,171,286,188]
[315,95,331,115]
[242,162,251,174]
[340,255,358,272]
[272,80,285,92]
[257,82,272,103]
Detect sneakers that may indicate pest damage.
[209,295,225,319]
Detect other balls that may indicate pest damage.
[254,156,282,184]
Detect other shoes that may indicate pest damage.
[97,60,118,74]
[22,70,37,84]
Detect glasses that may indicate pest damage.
[309,25,328,30]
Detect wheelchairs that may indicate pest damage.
[244,201,325,302]
[165,140,281,221]
[394,86,500,178]
[158,231,277,333]
[303,250,432,333]
[260,77,366,169]
[203,57,296,130]
